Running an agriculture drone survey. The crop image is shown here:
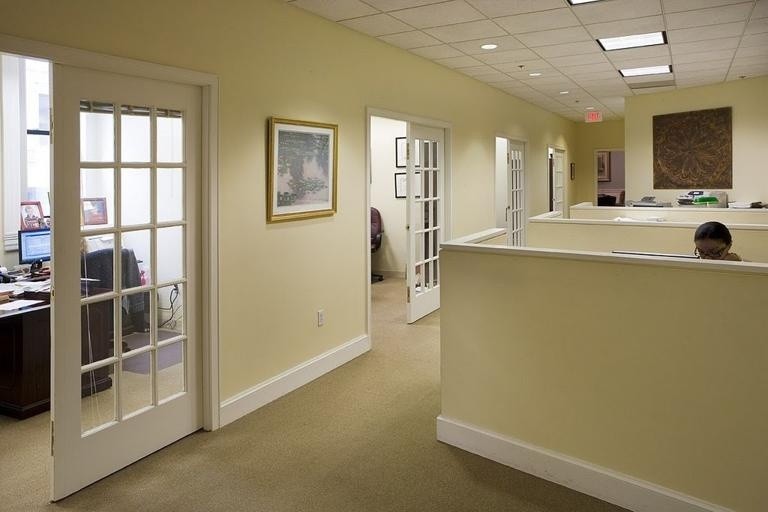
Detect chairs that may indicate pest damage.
[371,207,383,280]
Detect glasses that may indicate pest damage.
[694,245,728,257]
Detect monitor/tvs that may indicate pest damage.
[17,230,50,272]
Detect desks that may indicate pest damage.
[0,287,113,421]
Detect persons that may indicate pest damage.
[693,220,743,262]
[24,204,40,226]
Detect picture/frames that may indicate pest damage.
[598,151,611,182]
[395,171,421,198]
[82,198,107,225]
[20,201,45,231]
[395,136,419,168]
[571,164,576,180]
[265,117,339,223]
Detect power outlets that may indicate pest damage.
[318,310,324,326]
